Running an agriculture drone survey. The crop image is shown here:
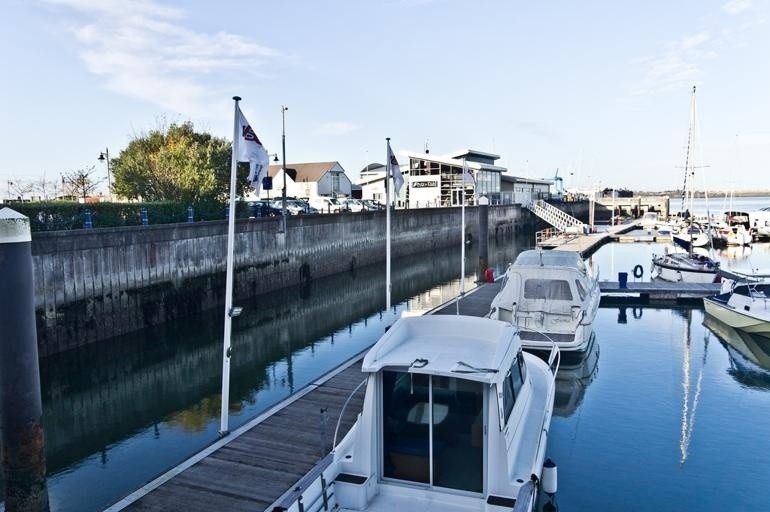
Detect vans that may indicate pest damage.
[243,196,382,218]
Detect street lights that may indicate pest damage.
[97,147,111,194]
[280,103,288,215]
[264,148,279,198]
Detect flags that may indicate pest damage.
[236,105,271,198]
[389,142,405,198]
[464,160,479,198]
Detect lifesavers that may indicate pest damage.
[634,265,643,278]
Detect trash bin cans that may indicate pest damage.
[618,272,628,288]
